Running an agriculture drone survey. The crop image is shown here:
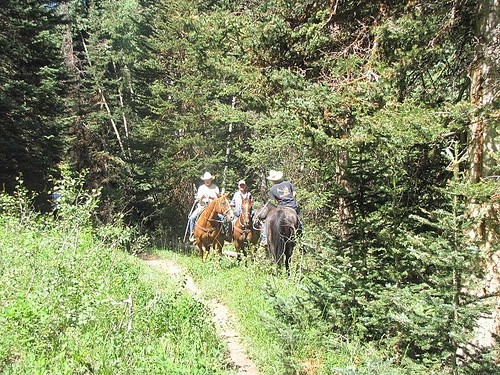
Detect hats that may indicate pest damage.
[238,180,245,185]
[200,172,216,180]
[265,170,283,182]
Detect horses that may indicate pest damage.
[265,207,298,278]
[192,193,234,262]
[233,194,261,267]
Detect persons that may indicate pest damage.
[189,171,225,242]
[222,180,256,243]
[259,170,303,247]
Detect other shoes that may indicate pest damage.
[189,234,195,242]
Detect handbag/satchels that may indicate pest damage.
[257,201,270,218]
